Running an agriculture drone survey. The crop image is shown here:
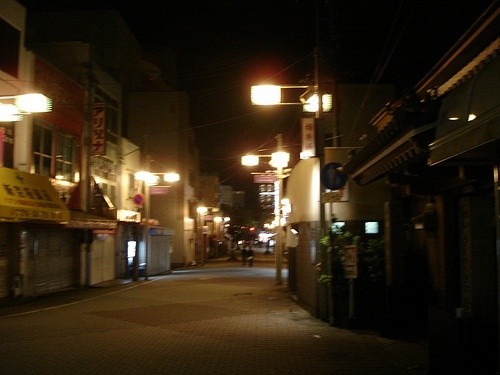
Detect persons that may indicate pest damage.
[241,244,248,267]
[247,246,255,266]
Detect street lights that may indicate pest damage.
[241,150,292,287]
[132,171,180,280]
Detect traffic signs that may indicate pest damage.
[322,192,342,204]
